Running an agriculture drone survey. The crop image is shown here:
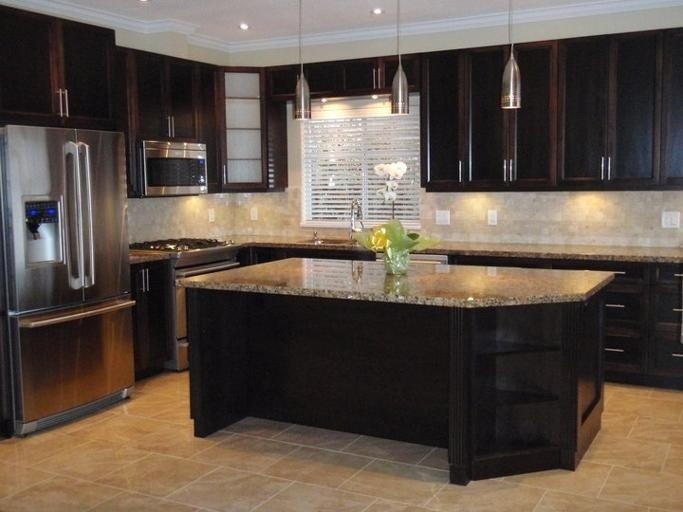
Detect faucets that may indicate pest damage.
[349,200,365,240]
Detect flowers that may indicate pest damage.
[351,161,441,272]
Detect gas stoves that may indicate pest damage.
[129,238,234,252]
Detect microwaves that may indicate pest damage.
[138,138,207,197]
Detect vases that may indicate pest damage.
[382,227,410,276]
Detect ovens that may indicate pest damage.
[159,262,241,371]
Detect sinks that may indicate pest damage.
[295,237,358,247]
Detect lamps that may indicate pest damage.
[293,0,410,119]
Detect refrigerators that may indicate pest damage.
[0,125,136,439]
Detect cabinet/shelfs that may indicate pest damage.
[420,50,466,192]
[466,29,682,192]
[267,53,421,108]
[130,263,167,382]
[0,4,118,130]
[218,65,289,193]
[588,262,683,391]
[467,308,569,483]
[129,46,200,143]
